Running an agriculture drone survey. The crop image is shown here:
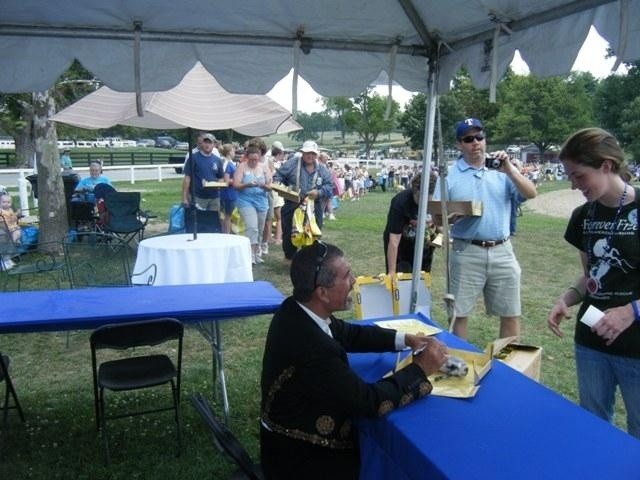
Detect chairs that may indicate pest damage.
[0,190,158,287]
[0,350,27,425]
[89,316,185,465]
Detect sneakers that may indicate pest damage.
[322,211,337,222]
[251,218,282,265]
[337,189,369,202]
[0,257,17,273]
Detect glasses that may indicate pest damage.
[312,239,328,287]
[461,134,485,144]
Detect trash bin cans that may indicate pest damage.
[25,174,82,242]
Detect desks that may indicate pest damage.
[130,232,255,287]
[0,281,287,450]
[335,310,640,480]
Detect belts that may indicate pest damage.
[452,234,510,248]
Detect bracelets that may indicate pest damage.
[631,300,640,319]
[569,287,582,302]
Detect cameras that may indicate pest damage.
[486,158,503,168]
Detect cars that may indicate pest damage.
[136,138,156,148]
[154,140,172,149]
[174,142,190,151]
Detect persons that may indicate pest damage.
[75,162,113,242]
[432,119,537,342]
[381,164,422,192]
[261,243,450,479]
[344,164,369,201]
[522,162,564,185]
[182,135,343,267]
[383,171,441,276]
[546,127,640,443]
[0,195,21,244]
[61,147,72,170]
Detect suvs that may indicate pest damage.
[360,150,384,164]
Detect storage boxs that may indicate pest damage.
[483,336,542,384]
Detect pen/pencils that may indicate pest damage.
[413,346,427,356]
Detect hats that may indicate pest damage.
[456,117,483,137]
[272,141,285,152]
[202,133,217,144]
[61,146,72,154]
[299,140,321,158]
